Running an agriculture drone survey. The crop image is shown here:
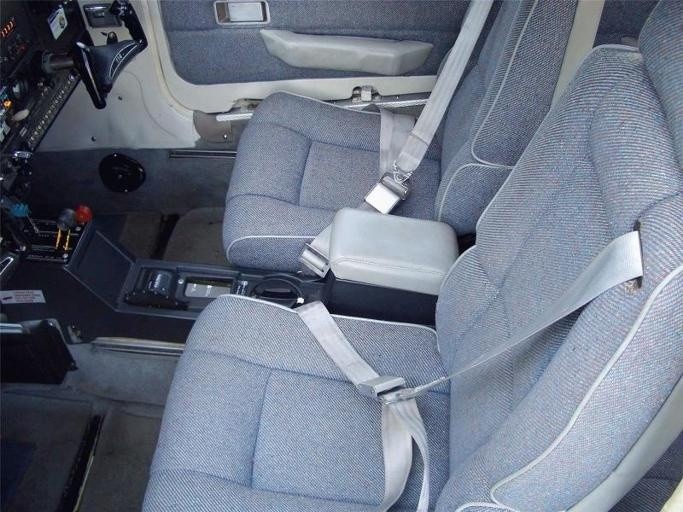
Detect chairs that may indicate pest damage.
[138,0,680,511]
[216,2,608,277]
